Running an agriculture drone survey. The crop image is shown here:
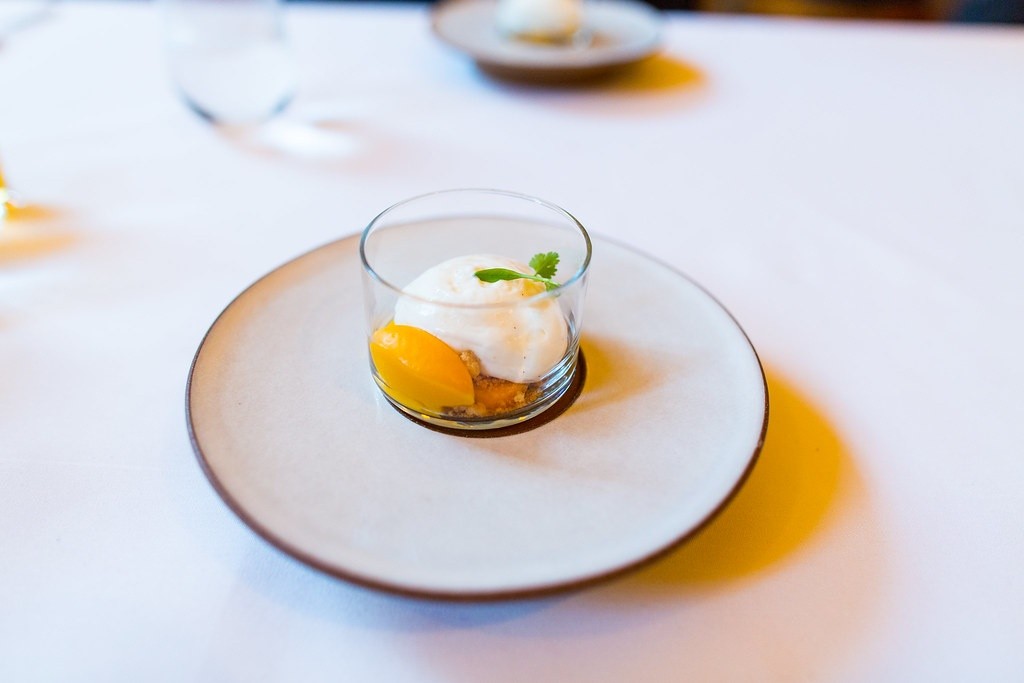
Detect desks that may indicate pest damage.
[0,0,1024,683]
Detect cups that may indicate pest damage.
[360,190,594,430]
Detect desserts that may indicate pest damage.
[393,255,568,382]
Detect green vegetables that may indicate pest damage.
[471,251,562,293]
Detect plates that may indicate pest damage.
[435,0,669,77]
[188,217,770,596]
